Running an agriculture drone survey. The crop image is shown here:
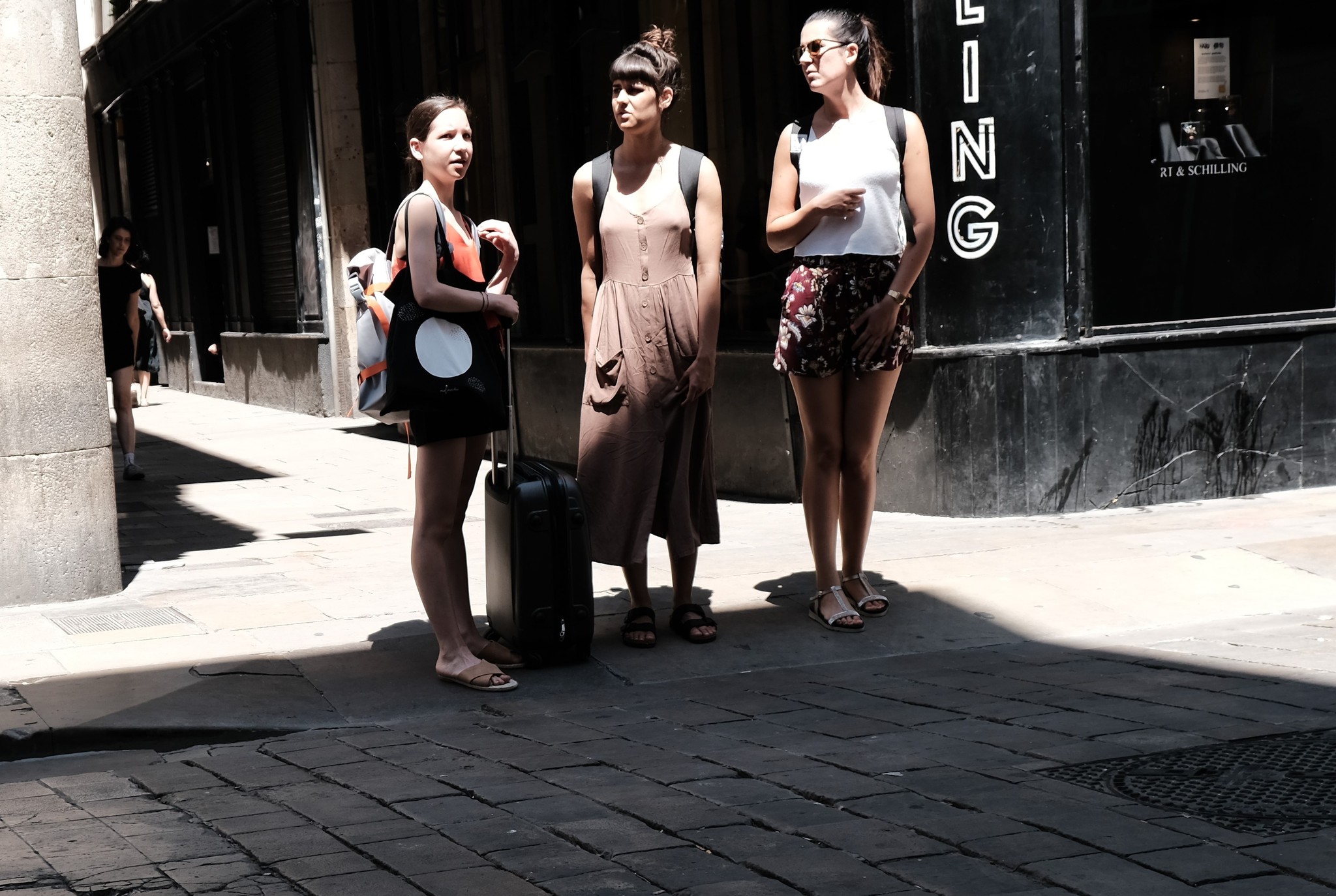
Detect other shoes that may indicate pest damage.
[131,385,139,408]
[140,399,149,406]
[126,466,145,480]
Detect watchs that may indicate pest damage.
[887,290,906,306]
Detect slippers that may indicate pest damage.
[480,639,523,670]
[441,660,520,691]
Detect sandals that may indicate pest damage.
[671,604,719,647]
[810,585,863,634]
[842,572,891,618]
[620,605,661,652]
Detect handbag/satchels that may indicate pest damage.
[380,261,508,426]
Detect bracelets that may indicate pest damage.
[478,290,489,314]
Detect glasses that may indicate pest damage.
[793,37,846,60]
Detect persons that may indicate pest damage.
[347,95,521,695]
[94,215,172,483]
[568,25,725,650]
[765,11,936,635]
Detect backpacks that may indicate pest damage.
[347,190,444,425]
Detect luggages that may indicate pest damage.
[485,320,595,668]
